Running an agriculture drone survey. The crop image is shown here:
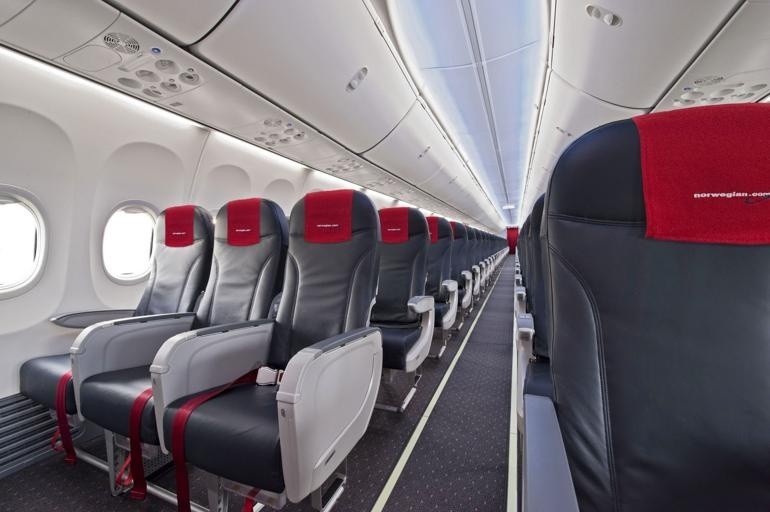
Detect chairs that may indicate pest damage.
[162,189,382,510]
[20,204,215,466]
[371,190,554,434]
[521,102,769,510]
[80,198,290,501]
